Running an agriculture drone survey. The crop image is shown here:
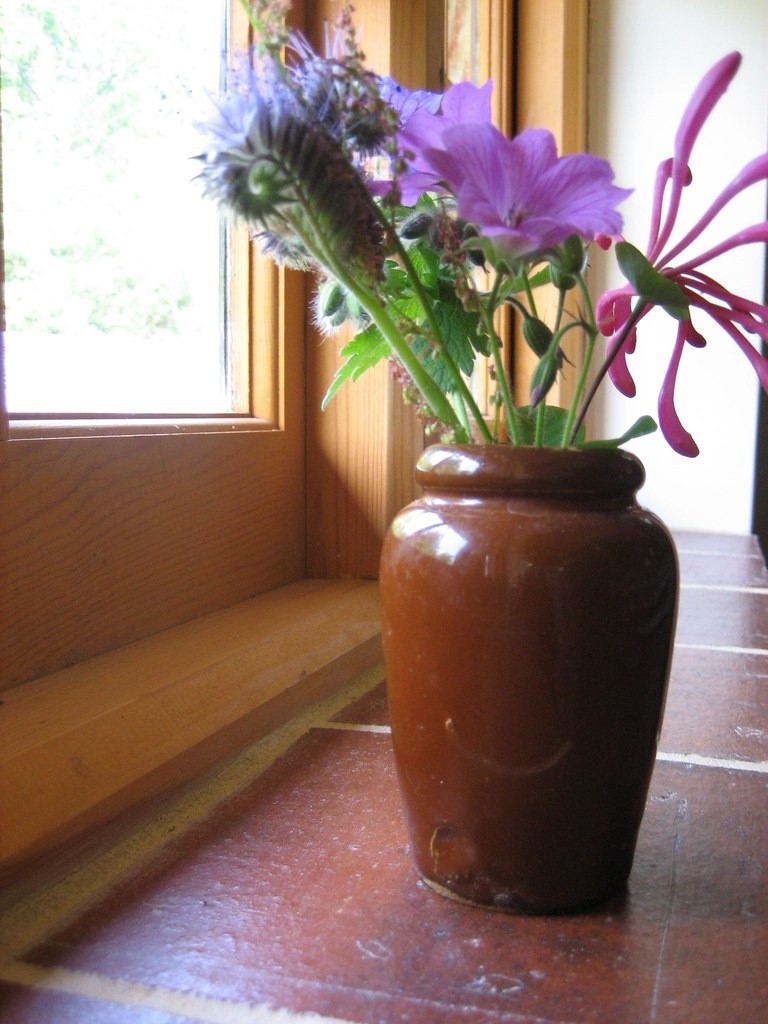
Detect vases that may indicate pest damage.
[379,440,680,913]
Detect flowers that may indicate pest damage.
[187,0,768,458]
